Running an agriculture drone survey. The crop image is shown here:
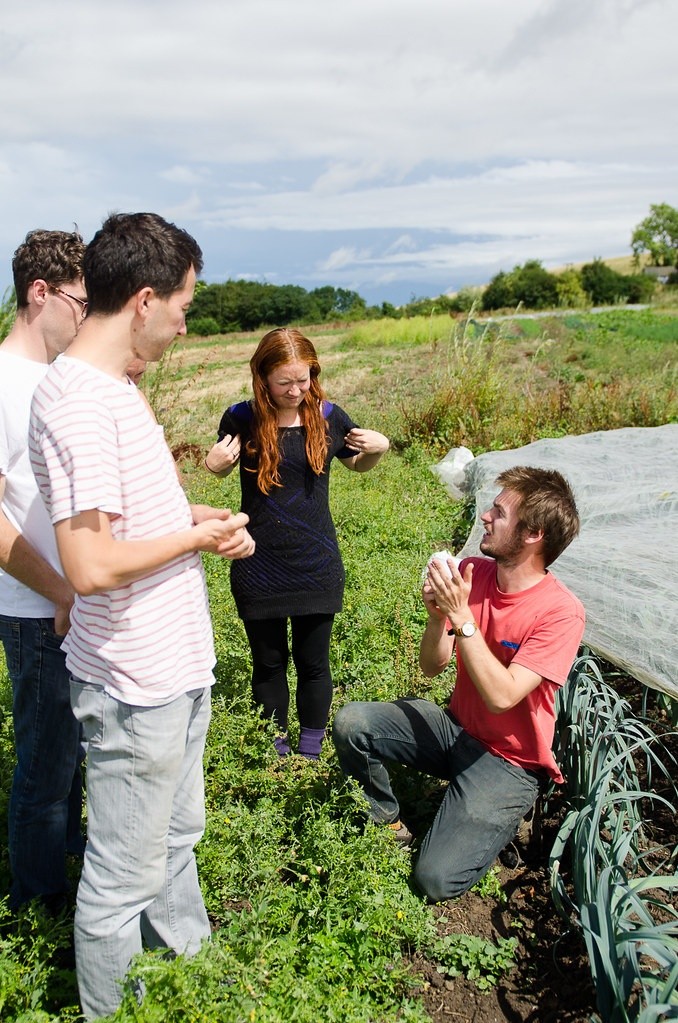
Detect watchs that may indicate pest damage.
[448,621,478,637]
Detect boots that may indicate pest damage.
[269,725,326,762]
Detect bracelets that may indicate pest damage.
[205,459,219,474]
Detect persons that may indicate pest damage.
[204,329,389,765]
[0,221,92,993]
[331,467,586,903]
[126,356,147,388]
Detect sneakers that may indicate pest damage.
[380,815,414,845]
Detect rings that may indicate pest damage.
[26,210,256,1021]
[232,451,237,457]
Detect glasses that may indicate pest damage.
[45,283,91,321]
